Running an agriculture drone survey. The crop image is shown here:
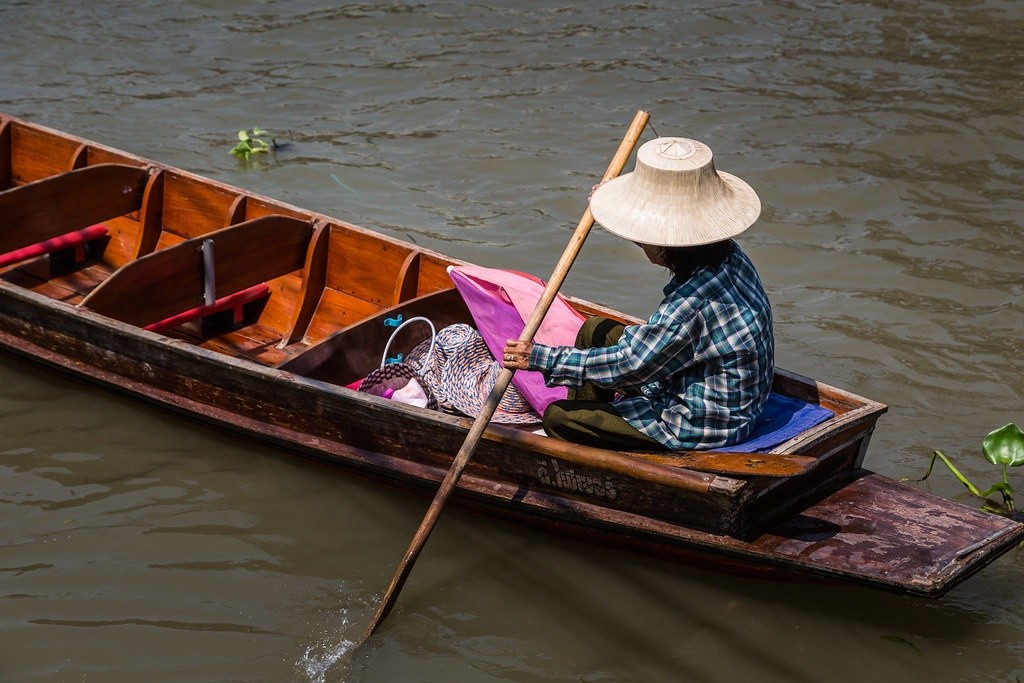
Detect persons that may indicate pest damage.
[500,137,775,452]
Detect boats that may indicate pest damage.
[0,111,1024,601]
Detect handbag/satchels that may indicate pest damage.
[357,316,439,415]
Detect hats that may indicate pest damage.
[404,322,483,415]
[440,335,543,423]
[588,137,761,245]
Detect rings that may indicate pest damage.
[512,355,514,360]
[508,355,511,360]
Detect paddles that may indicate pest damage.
[618,450,825,481]
[355,106,656,646]
